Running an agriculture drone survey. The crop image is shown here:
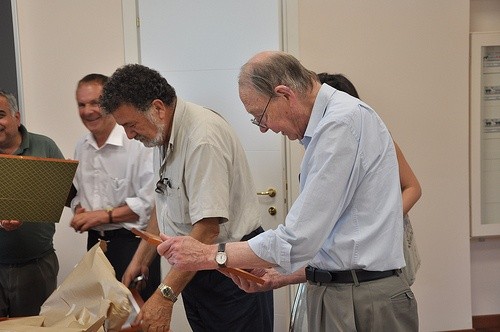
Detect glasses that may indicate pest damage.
[251,84,286,128]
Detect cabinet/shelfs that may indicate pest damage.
[469,30,500,240]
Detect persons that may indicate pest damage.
[69,73,160,303]
[0,92,78,318]
[157,50,419,332]
[317,73,423,287]
[98,64,275,332]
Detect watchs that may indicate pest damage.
[157,282,178,304]
[106,206,113,225]
[214,242,228,269]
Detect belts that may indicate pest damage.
[88,228,129,236]
[305,265,397,283]
